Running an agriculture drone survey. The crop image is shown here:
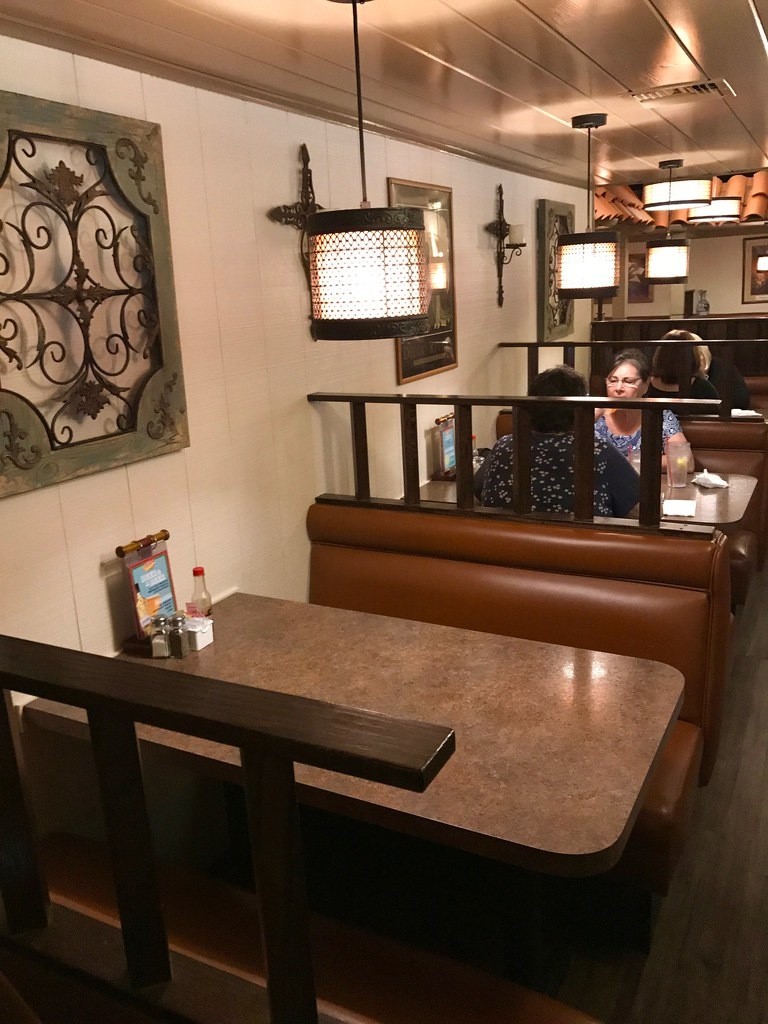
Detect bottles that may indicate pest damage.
[472,434,479,458]
[149,614,173,659]
[696,289,710,315]
[168,614,191,658]
[191,567,213,621]
[475,456,484,467]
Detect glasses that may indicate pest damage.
[604,376,644,386]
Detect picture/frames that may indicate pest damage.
[629,253,654,303]
[387,177,459,386]
[537,199,575,341]
[742,236,768,304]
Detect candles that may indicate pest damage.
[509,225,525,244]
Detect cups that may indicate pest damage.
[628,450,640,474]
[665,441,690,487]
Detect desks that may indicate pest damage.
[22,592,686,996]
[402,461,759,535]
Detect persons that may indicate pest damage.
[640,329,750,421]
[473,363,664,524]
[592,348,696,476]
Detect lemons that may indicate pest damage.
[677,457,686,470]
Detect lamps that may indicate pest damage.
[305,0,430,341]
[643,160,741,285]
[557,114,620,300]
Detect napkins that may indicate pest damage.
[662,500,695,516]
[691,468,729,488]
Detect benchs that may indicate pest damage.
[0,317,768,1024]
